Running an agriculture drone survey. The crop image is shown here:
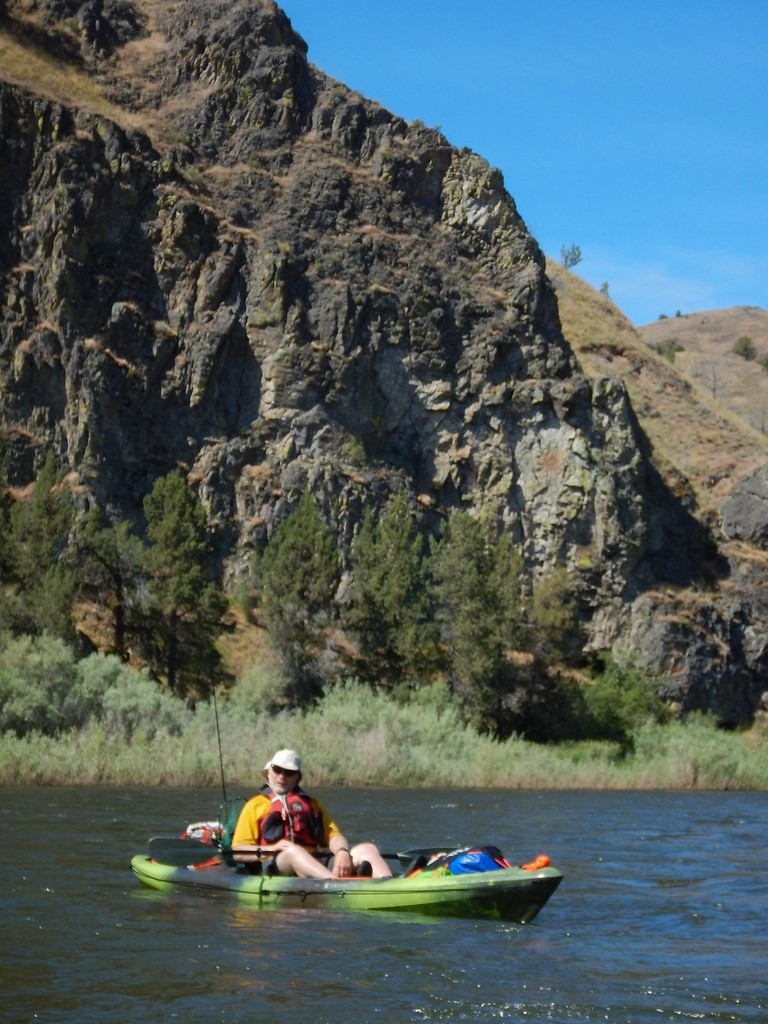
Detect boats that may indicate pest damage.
[128,796,564,926]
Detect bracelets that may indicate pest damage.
[337,848,349,853]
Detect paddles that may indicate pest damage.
[145,836,459,868]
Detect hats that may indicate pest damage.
[264,750,301,772]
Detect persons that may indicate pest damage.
[231,749,392,879]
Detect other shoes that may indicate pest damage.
[350,860,373,878]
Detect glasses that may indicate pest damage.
[271,764,298,777]
